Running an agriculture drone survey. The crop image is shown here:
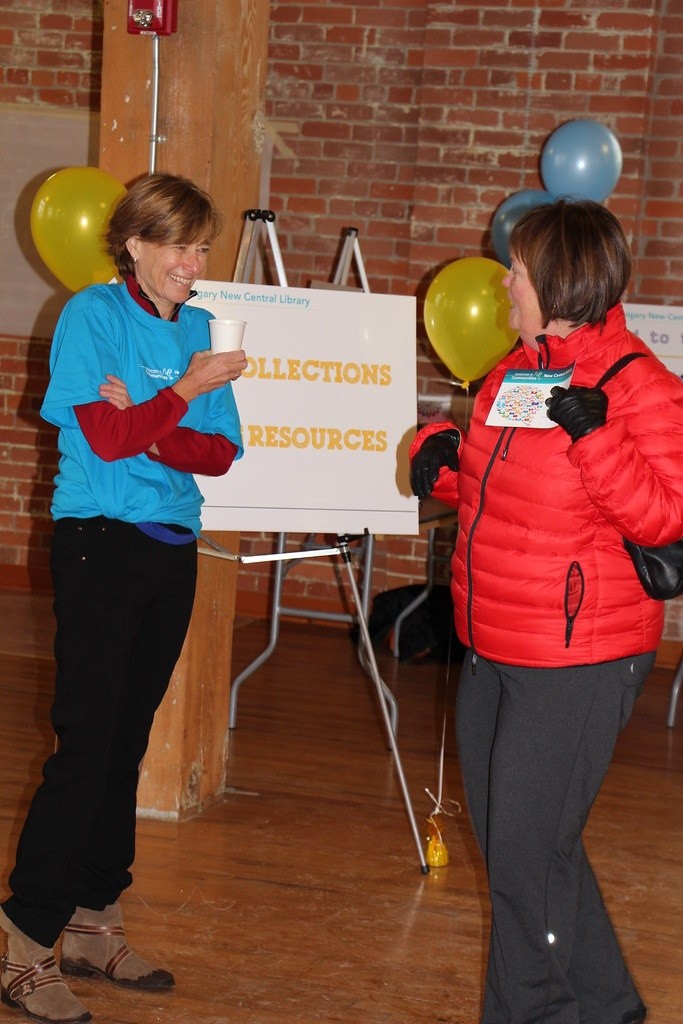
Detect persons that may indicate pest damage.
[409,198,682,1024]
[1,176,249,1024]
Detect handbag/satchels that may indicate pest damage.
[622,535,683,601]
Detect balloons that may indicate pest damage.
[30,165,135,296]
[422,257,522,391]
[538,120,625,208]
[487,187,557,272]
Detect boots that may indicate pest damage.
[59,899,176,989]
[0,905,92,1024]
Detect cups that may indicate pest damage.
[208,319,247,356]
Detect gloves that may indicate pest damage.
[409,429,461,500]
[545,384,609,445]
